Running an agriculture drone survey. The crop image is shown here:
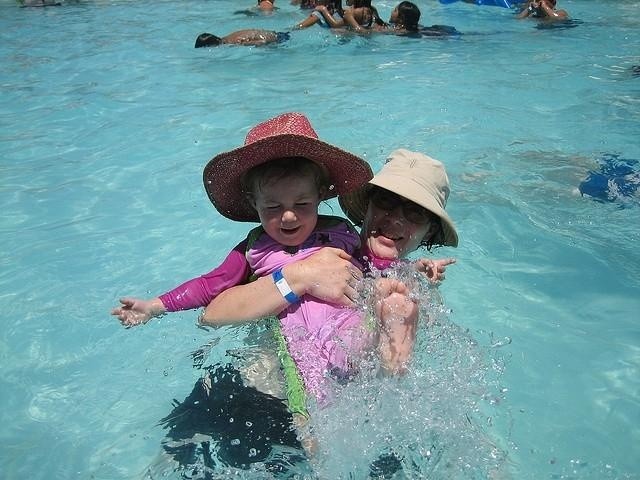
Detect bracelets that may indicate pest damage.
[272,267,300,306]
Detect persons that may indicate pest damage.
[194,0,421,46]
[196,149,457,480]
[517,0,568,17]
[105,136,361,480]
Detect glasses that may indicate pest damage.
[375,189,432,226]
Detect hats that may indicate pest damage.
[202,111,373,222]
[337,148,459,247]
